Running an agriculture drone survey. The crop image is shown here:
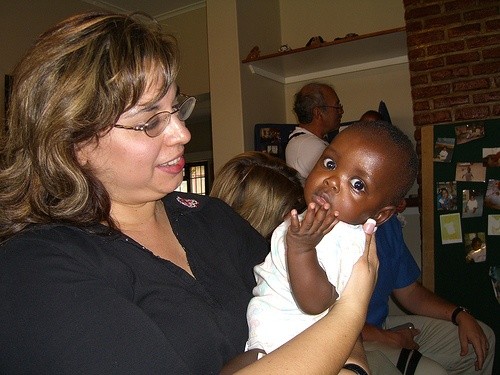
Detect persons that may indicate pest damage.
[0,6,495,375]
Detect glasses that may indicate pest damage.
[317,105,343,111]
[111,92,198,138]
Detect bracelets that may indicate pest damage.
[343,363,369,373]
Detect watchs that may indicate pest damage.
[451,306,471,324]
